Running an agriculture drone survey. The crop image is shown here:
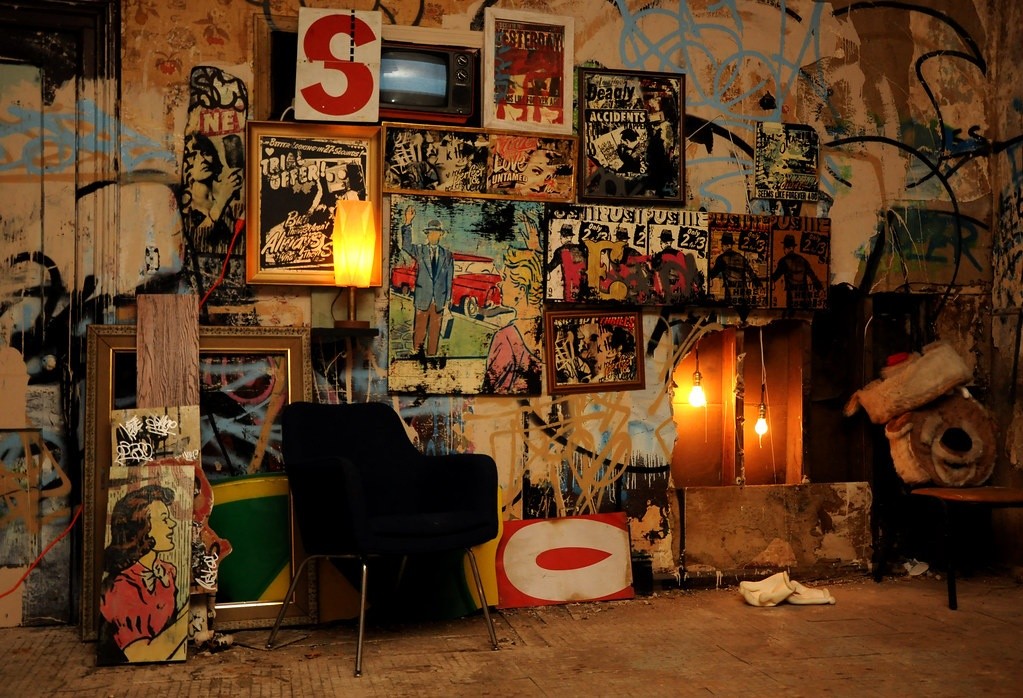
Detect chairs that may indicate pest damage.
[266,401,498,678]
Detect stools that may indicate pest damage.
[909,487,1023,611]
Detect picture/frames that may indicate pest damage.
[486,8,573,134]
[86,324,312,643]
[247,122,381,289]
[382,121,575,201]
[545,313,646,396]
[578,69,685,203]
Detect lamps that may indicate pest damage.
[333,200,377,286]
